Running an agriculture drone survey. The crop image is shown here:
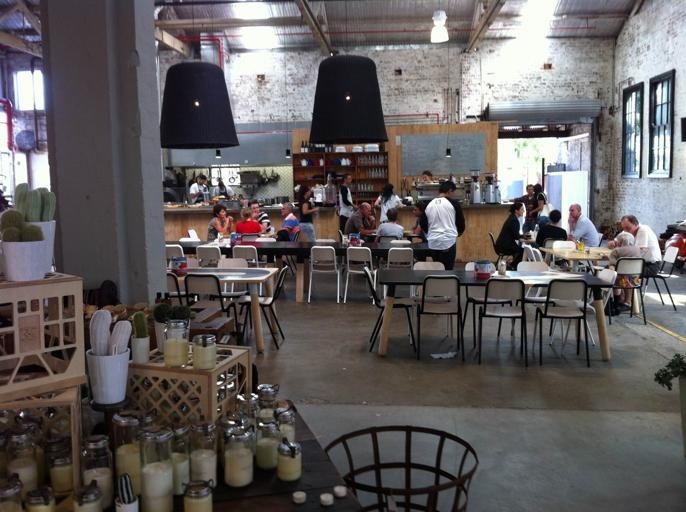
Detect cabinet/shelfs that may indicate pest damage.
[292,152,389,206]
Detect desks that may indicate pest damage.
[173,399,365,512]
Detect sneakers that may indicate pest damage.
[615,302,631,312]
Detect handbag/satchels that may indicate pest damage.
[537,203,556,217]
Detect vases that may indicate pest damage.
[86,348,130,405]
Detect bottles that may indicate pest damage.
[333,182,355,192]
[169,257,187,276]
[183,193,187,208]
[0,373,348,511]
[191,334,216,369]
[498,254,506,276]
[261,168,277,180]
[299,140,333,152]
[299,157,323,167]
[534,223,540,239]
[473,259,490,280]
[357,155,387,199]
[162,319,188,368]
[325,158,356,166]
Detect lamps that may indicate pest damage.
[310,0,387,144]
[161,1,239,148]
[430,0,450,44]
[285,0,291,159]
[445,0,452,159]
[215,148,221,159]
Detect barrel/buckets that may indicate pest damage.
[231,233,242,246]
[475,261,491,280]
[172,257,188,276]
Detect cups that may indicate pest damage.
[242,195,289,208]
[217,231,223,243]
[576,240,584,251]
[203,192,210,204]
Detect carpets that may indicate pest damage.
[168,264,685,413]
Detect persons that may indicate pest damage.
[494,182,602,274]
[204,197,431,263]
[605,231,644,317]
[188,174,209,204]
[373,183,405,224]
[607,215,664,311]
[420,180,466,271]
[422,170,434,181]
[212,181,237,200]
[297,184,320,242]
[338,173,359,241]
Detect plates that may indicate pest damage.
[187,203,202,208]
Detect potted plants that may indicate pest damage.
[0,208,46,282]
[130,311,151,364]
[151,303,170,354]
[13,183,57,275]
